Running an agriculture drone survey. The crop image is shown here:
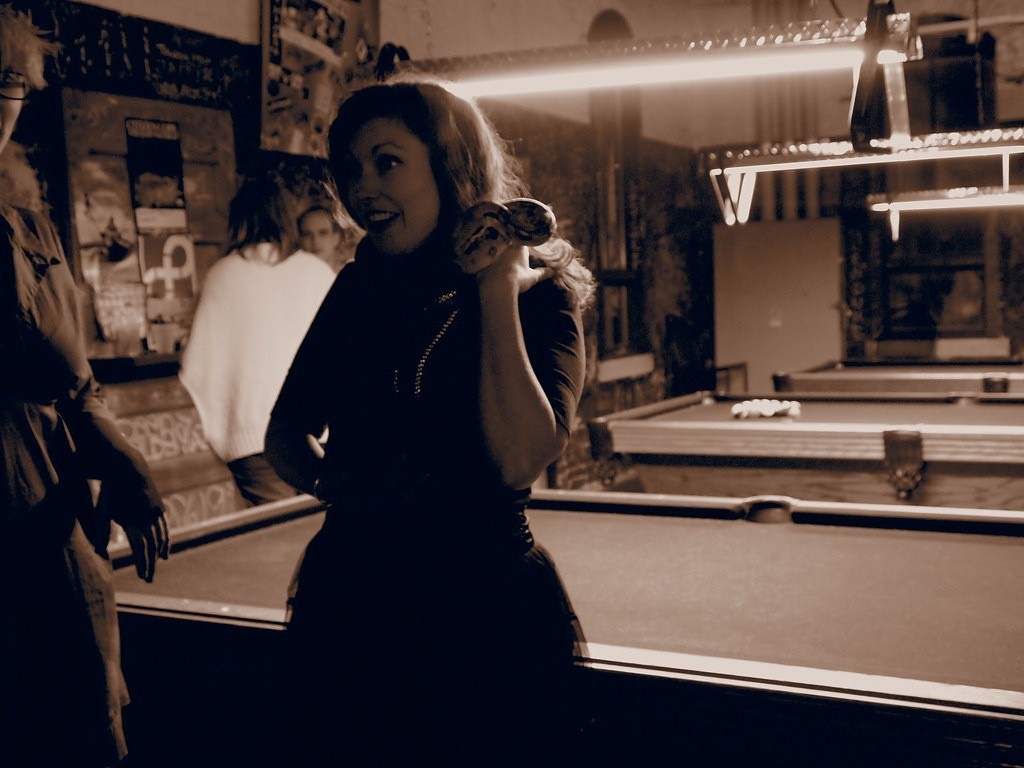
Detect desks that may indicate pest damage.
[99,349,1024,768]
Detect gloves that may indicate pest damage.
[79,416,170,584]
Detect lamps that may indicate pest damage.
[379,0,1024,241]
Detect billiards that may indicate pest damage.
[732,398,801,418]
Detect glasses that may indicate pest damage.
[0,71,32,100]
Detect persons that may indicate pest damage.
[179,168,339,509]
[293,203,346,267]
[0,10,171,768]
[262,81,592,768]
